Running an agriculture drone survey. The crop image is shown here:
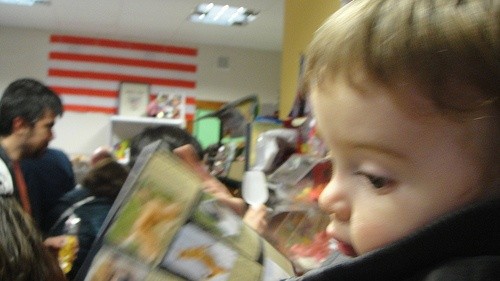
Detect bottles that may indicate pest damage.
[58,217,80,273]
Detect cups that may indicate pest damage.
[242,170,269,206]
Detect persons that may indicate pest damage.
[302,0,500,281]
[145,93,182,120]
[1,125,250,281]
[0,78,78,275]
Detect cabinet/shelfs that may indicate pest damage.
[108,115,194,165]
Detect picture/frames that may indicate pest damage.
[117,81,151,118]
